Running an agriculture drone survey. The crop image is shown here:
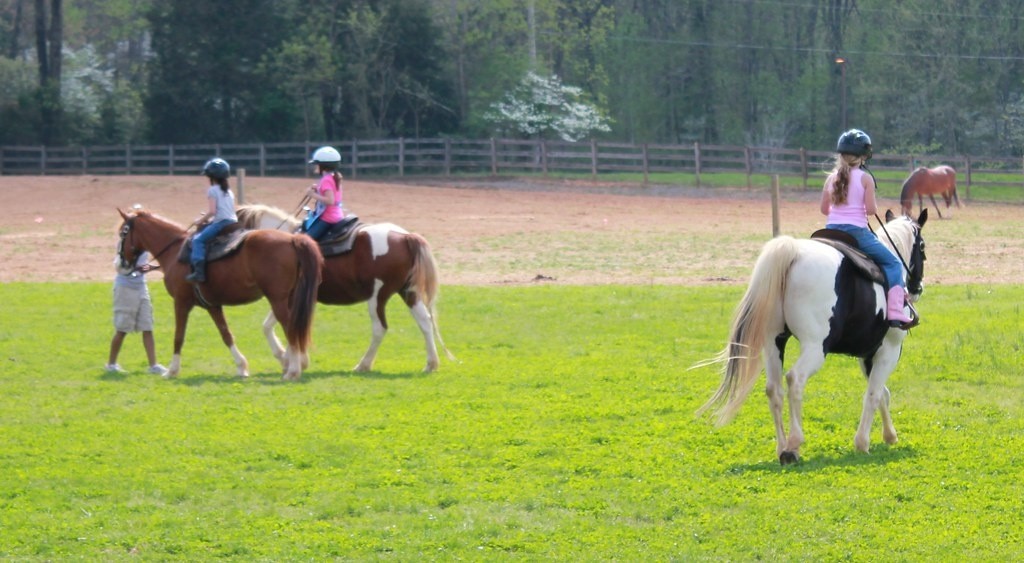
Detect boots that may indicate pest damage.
[887,284,913,327]
[185,260,206,281]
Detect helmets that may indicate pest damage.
[201,157,230,178]
[309,146,341,164]
[836,129,872,158]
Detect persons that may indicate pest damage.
[820,129,920,328]
[306,146,345,239]
[186,158,238,281]
[104,250,169,375]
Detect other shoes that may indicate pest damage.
[149,363,167,374]
[106,364,125,372]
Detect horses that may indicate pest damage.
[116,203,467,380]
[900,166,962,217]
[685,208,928,467]
